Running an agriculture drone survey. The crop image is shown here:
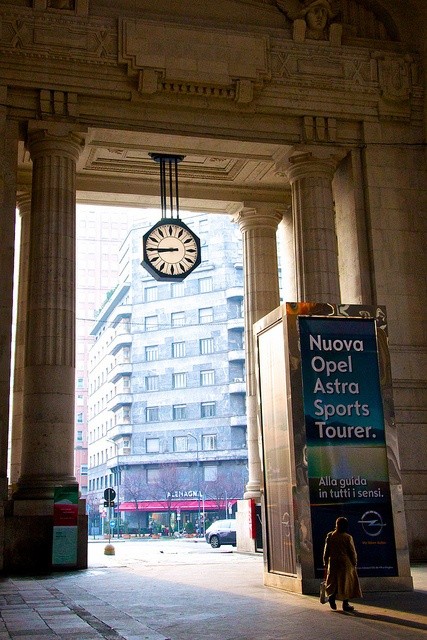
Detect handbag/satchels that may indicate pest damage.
[319,565,331,605]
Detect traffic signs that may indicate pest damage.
[110,520,114,527]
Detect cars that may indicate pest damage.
[204,520,236,549]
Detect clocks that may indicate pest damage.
[136,150,208,286]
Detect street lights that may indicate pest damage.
[187,433,202,537]
[106,439,120,538]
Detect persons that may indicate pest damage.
[323,517,363,612]
